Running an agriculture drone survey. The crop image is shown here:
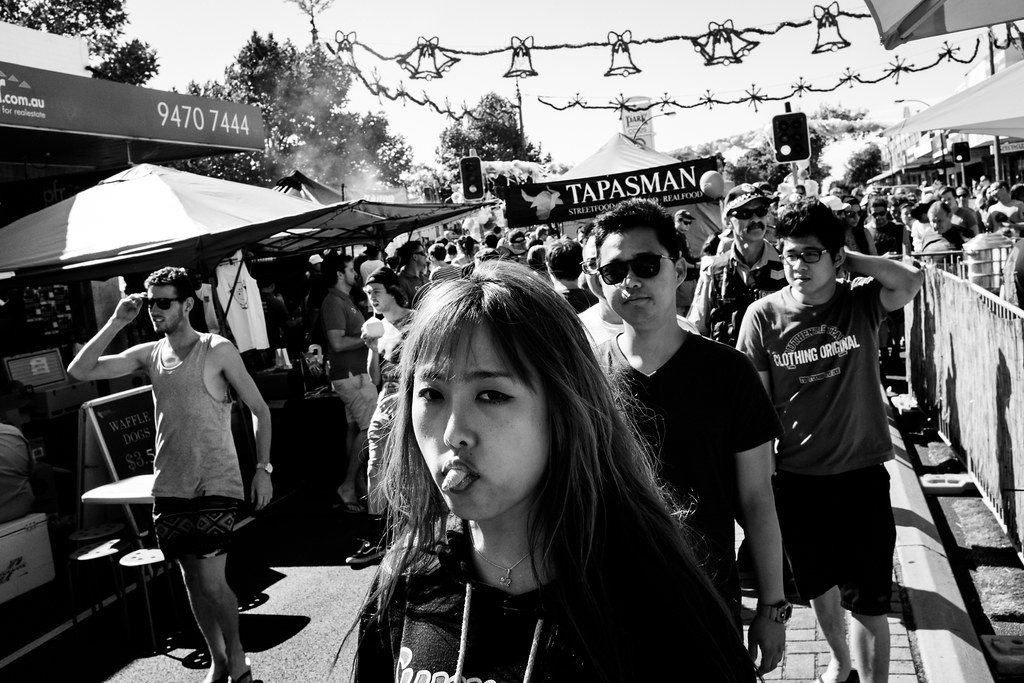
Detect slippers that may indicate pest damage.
[818,667,857,683]
[210,656,253,683]
[332,501,366,514]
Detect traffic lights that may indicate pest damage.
[773,112,811,163]
[459,156,485,201]
[952,141,971,164]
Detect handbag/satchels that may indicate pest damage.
[738,536,812,608]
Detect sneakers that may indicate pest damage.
[345,537,386,564]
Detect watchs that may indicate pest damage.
[256,462,273,473]
[757,601,793,624]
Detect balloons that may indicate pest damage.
[698,170,724,198]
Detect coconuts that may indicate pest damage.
[362,316,384,339]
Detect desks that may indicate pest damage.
[81,469,160,510]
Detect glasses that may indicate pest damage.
[843,210,862,218]
[142,297,180,310]
[729,205,768,220]
[778,249,827,265]
[596,253,674,285]
[579,260,598,275]
[682,220,692,225]
[956,193,968,198]
[413,250,425,256]
[872,211,886,217]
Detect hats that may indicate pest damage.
[818,195,851,212]
[365,267,400,288]
[753,181,772,192]
[675,210,696,220]
[724,183,773,218]
[508,230,526,243]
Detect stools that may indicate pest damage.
[69,520,124,552]
[66,540,137,637]
[120,547,184,649]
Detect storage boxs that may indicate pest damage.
[0,511,56,606]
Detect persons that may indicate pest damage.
[590,198,787,677]
[735,196,924,683]
[328,259,756,683]
[0,180,1024,625]
[67,264,273,683]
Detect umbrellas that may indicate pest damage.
[246,170,497,266]
[0,163,349,460]
[876,59,1024,141]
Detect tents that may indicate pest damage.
[555,133,682,180]
[864,0,1024,50]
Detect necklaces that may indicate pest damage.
[473,536,543,587]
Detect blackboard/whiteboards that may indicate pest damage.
[75,385,155,537]
